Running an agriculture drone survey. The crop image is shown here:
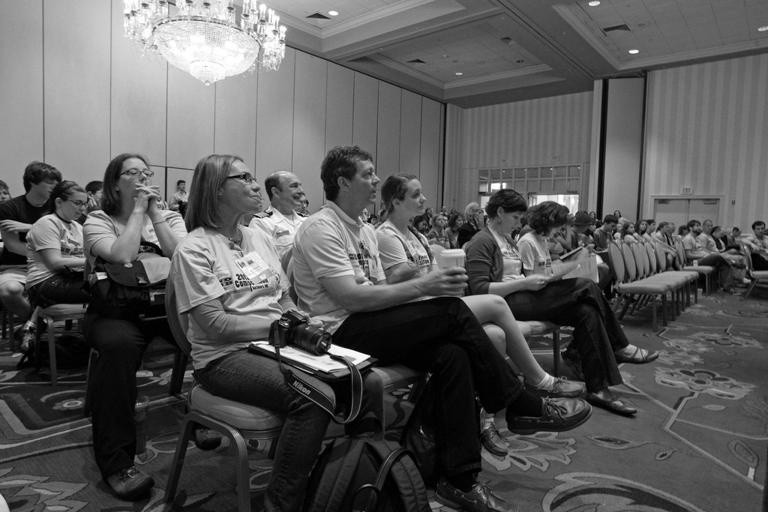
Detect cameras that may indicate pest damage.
[269,310,334,356]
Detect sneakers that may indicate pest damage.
[190,421,221,444]
[561,350,585,381]
[106,466,154,498]
[13,325,37,353]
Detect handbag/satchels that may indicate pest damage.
[15,335,100,371]
[403,374,481,491]
[88,241,171,321]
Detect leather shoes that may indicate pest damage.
[586,388,637,415]
[480,375,591,456]
[433,479,516,511]
[614,346,658,364]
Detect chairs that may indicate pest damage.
[607,231,767,331]
[1,223,597,511]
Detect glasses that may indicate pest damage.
[226,173,257,182]
[69,199,89,207]
[119,168,153,177]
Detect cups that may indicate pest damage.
[441,249,465,294]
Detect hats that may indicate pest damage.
[569,210,596,226]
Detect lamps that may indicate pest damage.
[122,0,287,84]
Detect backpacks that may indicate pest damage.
[299,411,432,511]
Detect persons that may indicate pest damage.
[371,171,589,457]
[80,154,195,502]
[653,217,767,296]
[250,171,314,268]
[1,152,188,346]
[465,189,660,417]
[164,154,383,510]
